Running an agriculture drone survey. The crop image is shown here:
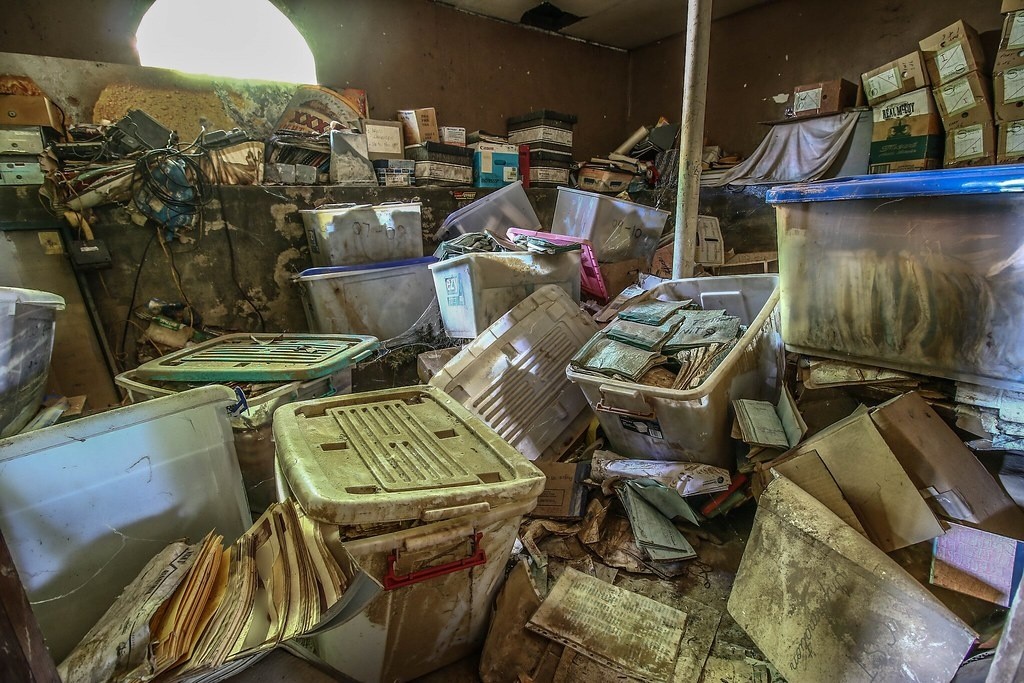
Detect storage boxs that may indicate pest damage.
[1,107,1023,682]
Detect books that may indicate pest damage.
[47,140,1005,682]
